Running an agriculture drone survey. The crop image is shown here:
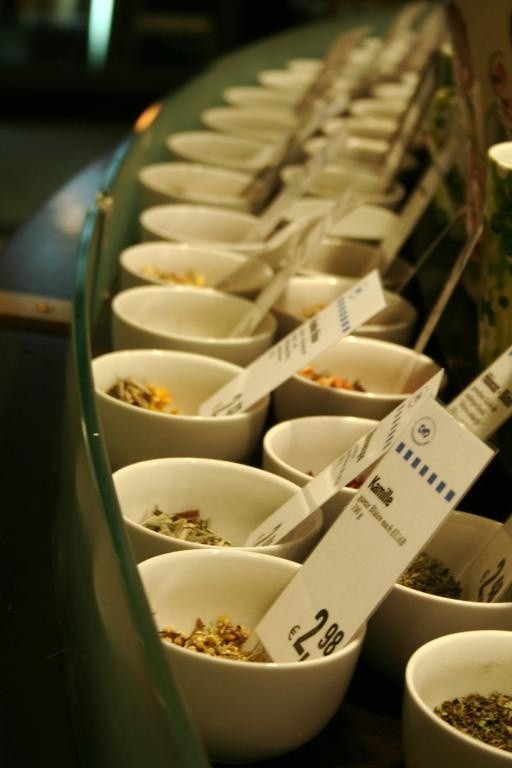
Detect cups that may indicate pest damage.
[478,143,512,366]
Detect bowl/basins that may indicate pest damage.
[88,25,511,760]
